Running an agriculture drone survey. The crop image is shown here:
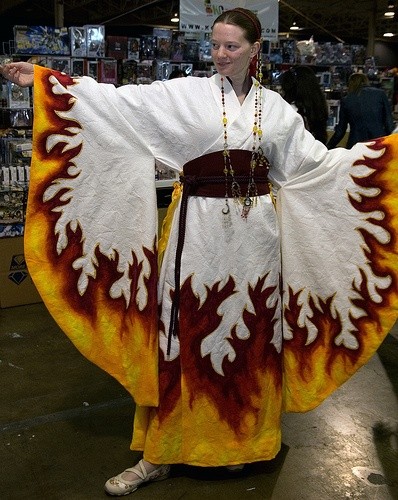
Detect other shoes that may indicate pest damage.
[104,459,171,496]
[226,465,244,471]
